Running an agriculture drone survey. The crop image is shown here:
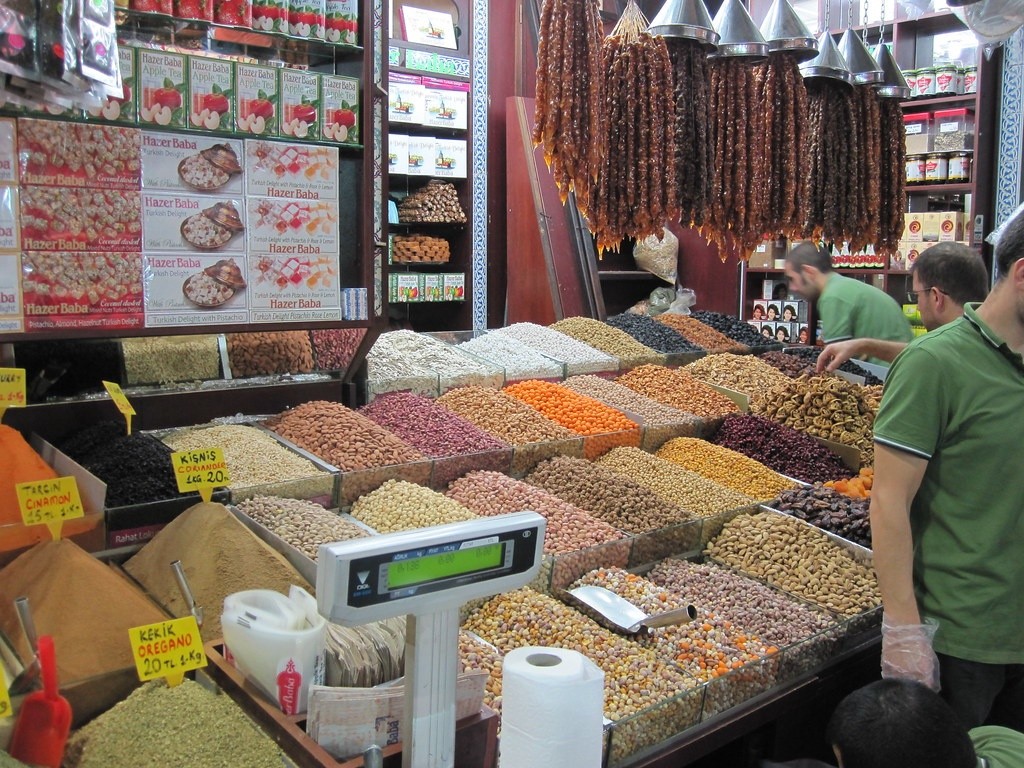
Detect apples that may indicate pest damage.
[42,75,358,143]
[250,0,358,44]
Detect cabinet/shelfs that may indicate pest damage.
[737,8,996,346]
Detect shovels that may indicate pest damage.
[169,559,204,630]
[3,634,75,768]
[558,584,697,634]
[7,596,43,696]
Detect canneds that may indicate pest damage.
[901,64,978,101]
[906,150,974,187]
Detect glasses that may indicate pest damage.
[907,287,949,302]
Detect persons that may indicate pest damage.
[869,211,1024,733]
[785,239,913,368]
[753,304,766,320]
[831,679,1024,768]
[767,304,780,321]
[817,241,989,372]
[796,327,808,344]
[761,325,773,339]
[775,326,789,342]
[784,305,797,321]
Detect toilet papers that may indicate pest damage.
[498,643,606,767]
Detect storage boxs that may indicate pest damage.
[934,108,974,150]
[888,210,965,270]
[901,112,934,152]
[0,116,343,333]
[0,316,918,768]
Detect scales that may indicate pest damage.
[314,508,550,768]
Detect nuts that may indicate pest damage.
[221,308,883,765]
